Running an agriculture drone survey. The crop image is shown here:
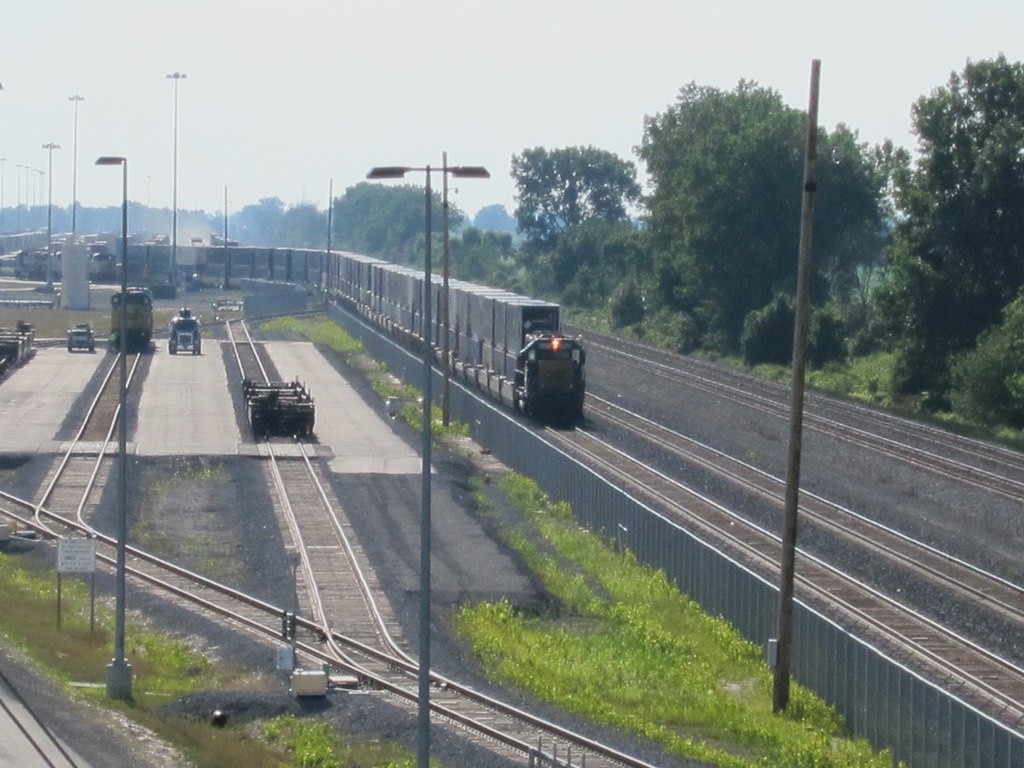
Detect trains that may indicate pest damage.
[0,240,118,286]
[110,287,153,350]
[129,244,585,421]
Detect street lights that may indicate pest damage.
[365,165,490,426]
[0,72,188,285]
[94,155,129,664]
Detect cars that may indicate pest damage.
[67,323,94,351]
[168,308,200,354]
